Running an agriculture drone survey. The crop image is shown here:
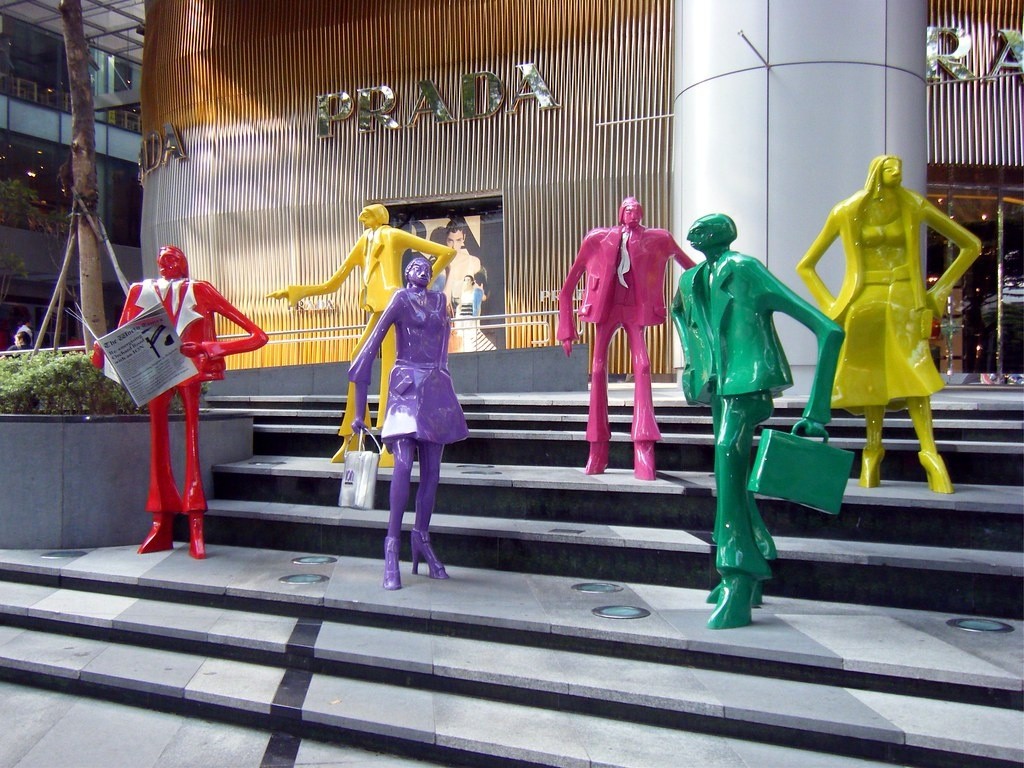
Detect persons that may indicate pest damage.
[795,156,982,492]
[669,215,846,630]
[347,258,472,589]
[6,319,34,351]
[452,272,487,353]
[265,203,457,467]
[557,198,697,481]
[93,245,269,559]
[440,220,490,320]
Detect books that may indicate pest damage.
[64,302,199,408]
